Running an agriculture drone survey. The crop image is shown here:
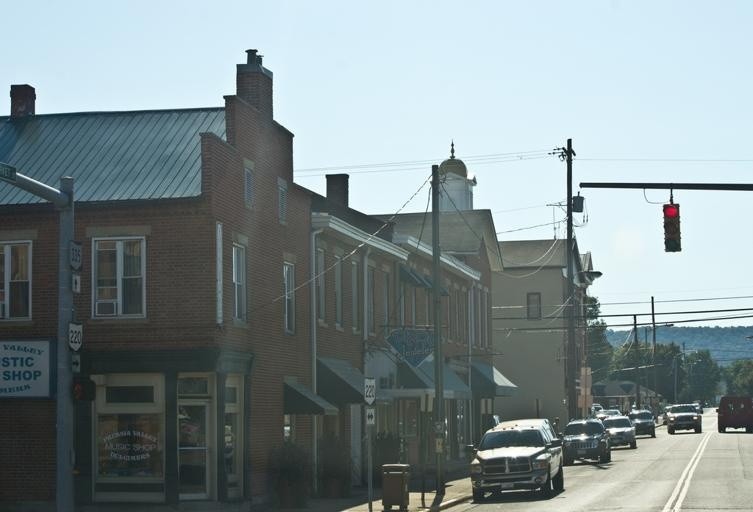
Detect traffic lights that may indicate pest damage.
[662,204,682,253]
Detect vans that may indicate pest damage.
[715,396,752,432]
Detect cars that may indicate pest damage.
[593,399,703,449]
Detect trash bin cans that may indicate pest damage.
[382,464,410,512]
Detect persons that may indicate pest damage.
[552,416,560,434]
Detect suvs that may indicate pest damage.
[465,418,566,501]
[561,419,612,465]
